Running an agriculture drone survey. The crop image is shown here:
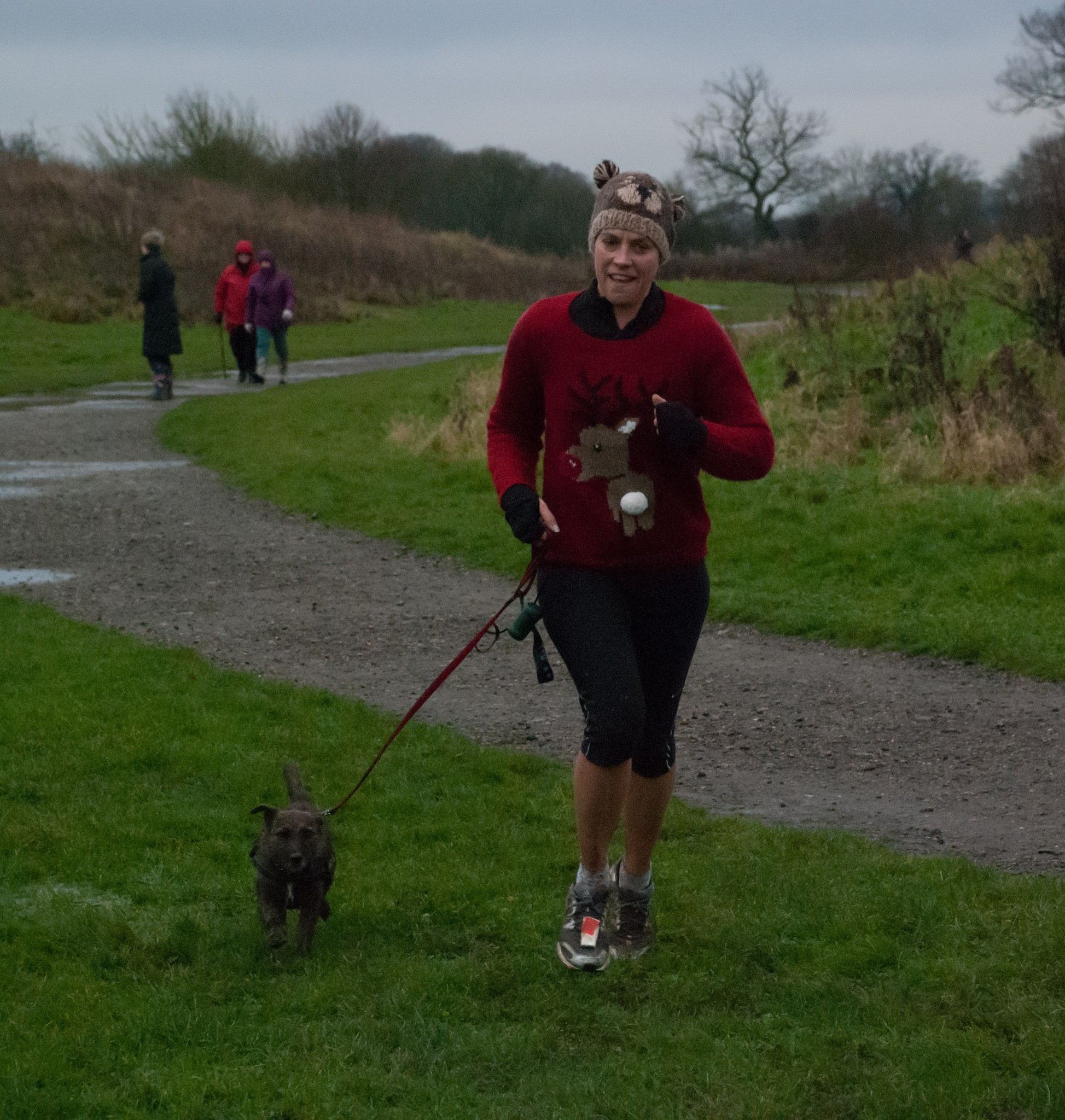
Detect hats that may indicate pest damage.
[588,160,686,266]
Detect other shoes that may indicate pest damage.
[144,358,295,404]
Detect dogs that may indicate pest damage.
[249,765,336,954]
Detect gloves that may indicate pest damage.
[214,313,222,326]
[243,321,254,334]
[280,309,293,322]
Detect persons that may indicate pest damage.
[214,240,261,384]
[133,229,184,402]
[484,158,778,972]
[242,248,296,385]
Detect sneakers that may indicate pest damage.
[557,872,613,971]
[608,855,655,961]
